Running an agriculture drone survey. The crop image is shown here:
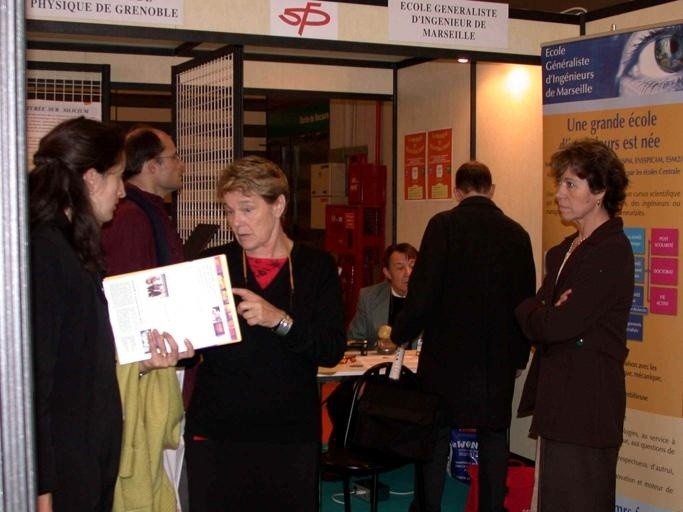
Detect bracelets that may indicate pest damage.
[272,314,293,338]
[139,371,145,378]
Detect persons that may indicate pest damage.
[615,24,683,97]
[513,139,635,512]
[390,161,537,512]
[175,155,346,512]
[101,121,201,512]
[28,117,195,512]
[327,244,423,481]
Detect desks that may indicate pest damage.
[317,349,418,377]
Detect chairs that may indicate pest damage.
[317,362,421,512]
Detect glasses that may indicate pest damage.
[156,153,180,161]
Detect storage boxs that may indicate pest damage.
[311,161,387,334]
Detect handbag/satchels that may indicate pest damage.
[466,465,536,512]
[449,430,479,481]
